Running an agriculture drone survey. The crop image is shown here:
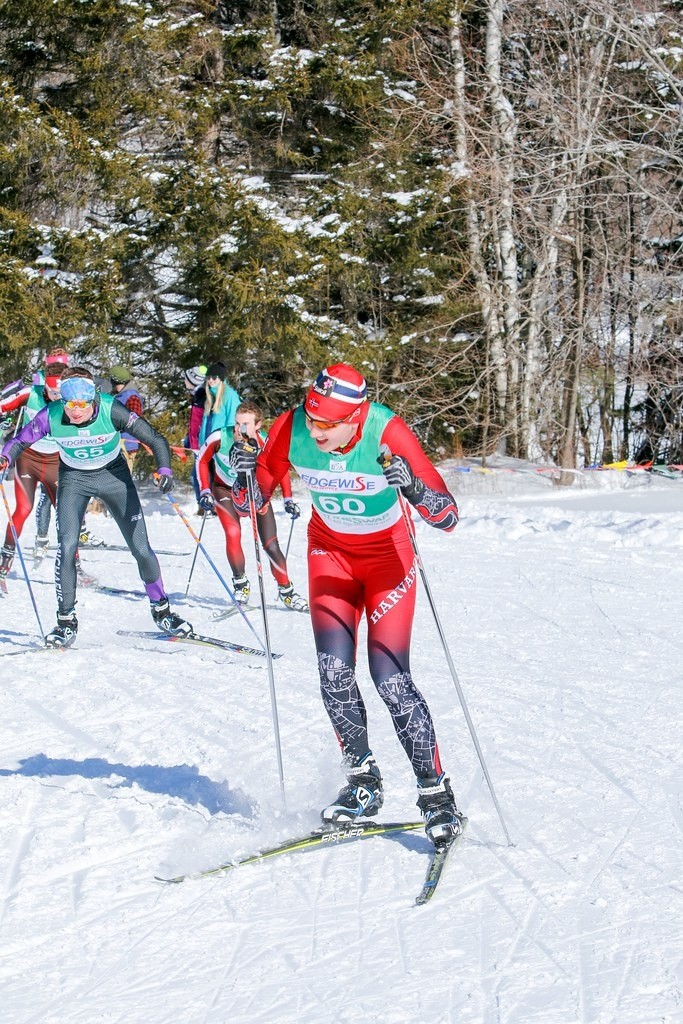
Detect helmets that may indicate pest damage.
[107,367,129,384]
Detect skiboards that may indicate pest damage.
[0,574,146,601]
[0,629,286,662]
[15,543,192,568]
[152,816,466,905]
[212,605,311,621]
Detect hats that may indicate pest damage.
[185,365,208,386]
[308,365,369,425]
[205,362,226,381]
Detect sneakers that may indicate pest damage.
[45,610,78,646]
[317,751,385,827]
[417,772,462,849]
[74,553,98,587]
[150,597,194,638]
[0,549,16,594]
[277,582,308,612]
[80,530,104,549]
[233,574,251,603]
[33,537,51,560]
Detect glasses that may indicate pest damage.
[300,399,365,430]
[206,375,219,381]
[46,384,59,396]
[58,397,96,411]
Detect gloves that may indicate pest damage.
[199,493,216,512]
[376,452,424,506]
[0,456,9,483]
[229,443,257,486]
[152,474,175,494]
[285,499,301,521]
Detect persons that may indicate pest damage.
[185,361,241,516]
[0,349,143,586]
[230,362,460,845]
[0,377,193,645]
[195,403,308,611]
